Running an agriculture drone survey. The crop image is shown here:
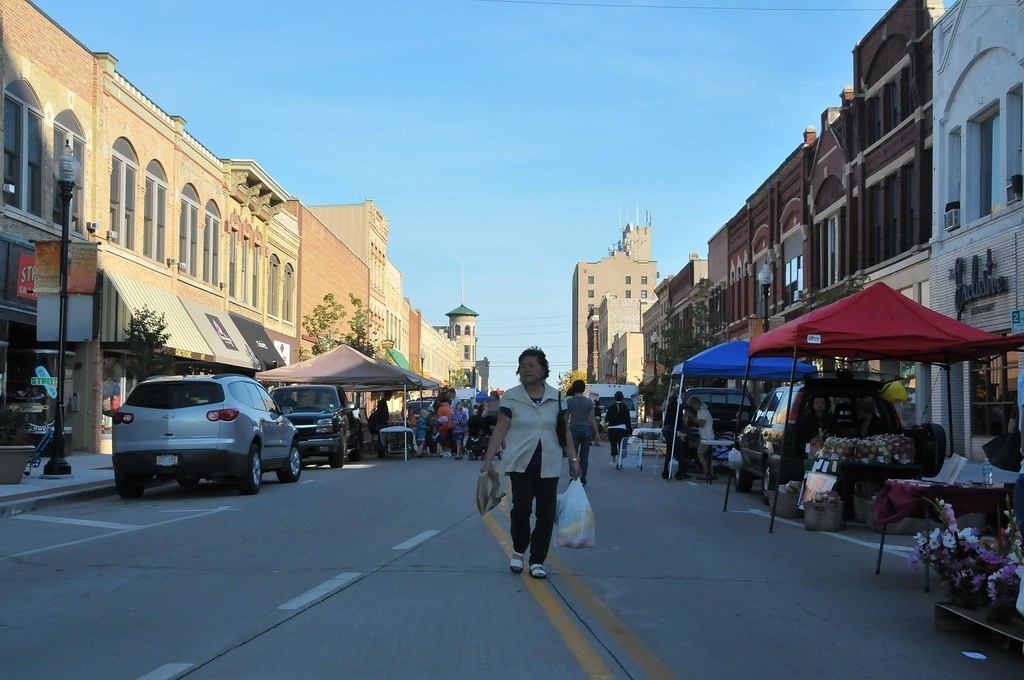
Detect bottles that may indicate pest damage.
[728,448,743,469]
[981,458,992,484]
[999,537,1006,557]
[668,458,679,474]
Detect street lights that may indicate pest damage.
[420,349,426,408]
[614,357,619,384]
[44,139,80,475]
[447,362,453,386]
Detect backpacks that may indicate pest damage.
[368,411,379,433]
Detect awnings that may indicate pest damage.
[102,270,286,370]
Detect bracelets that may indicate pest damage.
[569,458,578,463]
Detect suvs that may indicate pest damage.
[401,396,437,421]
[683,388,757,433]
[270,383,363,468]
[735,371,904,505]
[111,374,301,498]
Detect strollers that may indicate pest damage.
[465,414,503,460]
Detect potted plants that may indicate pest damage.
[0,402,36,484]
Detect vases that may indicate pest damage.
[943,581,1017,623]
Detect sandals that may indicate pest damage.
[509,550,523,573]
[529,563,546,578]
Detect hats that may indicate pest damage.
[438,415,448,423]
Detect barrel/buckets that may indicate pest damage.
[803,502,842,531]
[853,495,875,530]
[764,490,803,518]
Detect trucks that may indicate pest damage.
[583,383,640,432]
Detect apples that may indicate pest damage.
[824,435,915,465]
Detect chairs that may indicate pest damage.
[834,403,853,429]
[370,433,379,451]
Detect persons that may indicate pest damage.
[662,387,691,479]
[566,380,602,485]
[480,349,581,577]
[377,400,389,457]
[408,388,500,459]
[803,395,841,444]
[605,391,632,465]
[687,396,714,479]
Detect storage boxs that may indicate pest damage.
[764,490,920,535]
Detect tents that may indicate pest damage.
[255,344,438,460]
[654,340,818,479]
[723,281,1024,533]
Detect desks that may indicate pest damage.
[870,479,1015,593]
[701,439,735,485]
[616,427,663,474]
[25,431,74,456]
[380,426,419,458]
[802,459,923,531]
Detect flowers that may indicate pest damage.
[907,497,1024,600]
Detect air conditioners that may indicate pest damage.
[943,209,959,230]
[793,290,803,302]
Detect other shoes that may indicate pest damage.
[662,472,672,478]
[455,455,461,459]
[443,454,451,457]
[440,452,444,456]
[581,478,586,484]
[697,476,707,480]
[677,474,692,481]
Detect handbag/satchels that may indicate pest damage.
[712,448,730,460]
[555,391,568,447]
[555,476,595,547]
[689,440,698,448]
[728,447,744,469]
[603,422,609,429]
